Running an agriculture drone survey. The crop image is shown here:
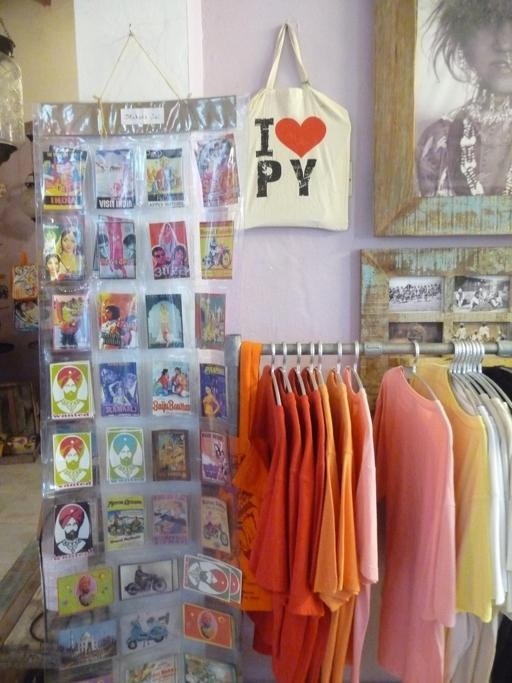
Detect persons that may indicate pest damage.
[415,0,510,195]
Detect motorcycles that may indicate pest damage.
[202,243,231,270]
[125,574,168,596]
[202,522,229,546]
[126,615,166,650]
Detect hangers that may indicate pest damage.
[264,341,512,443]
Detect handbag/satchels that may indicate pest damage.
[243,21,352,232]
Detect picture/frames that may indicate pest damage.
[360,247,512,341]
[373,0,512,236]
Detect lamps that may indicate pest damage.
[0,16,26,169]
